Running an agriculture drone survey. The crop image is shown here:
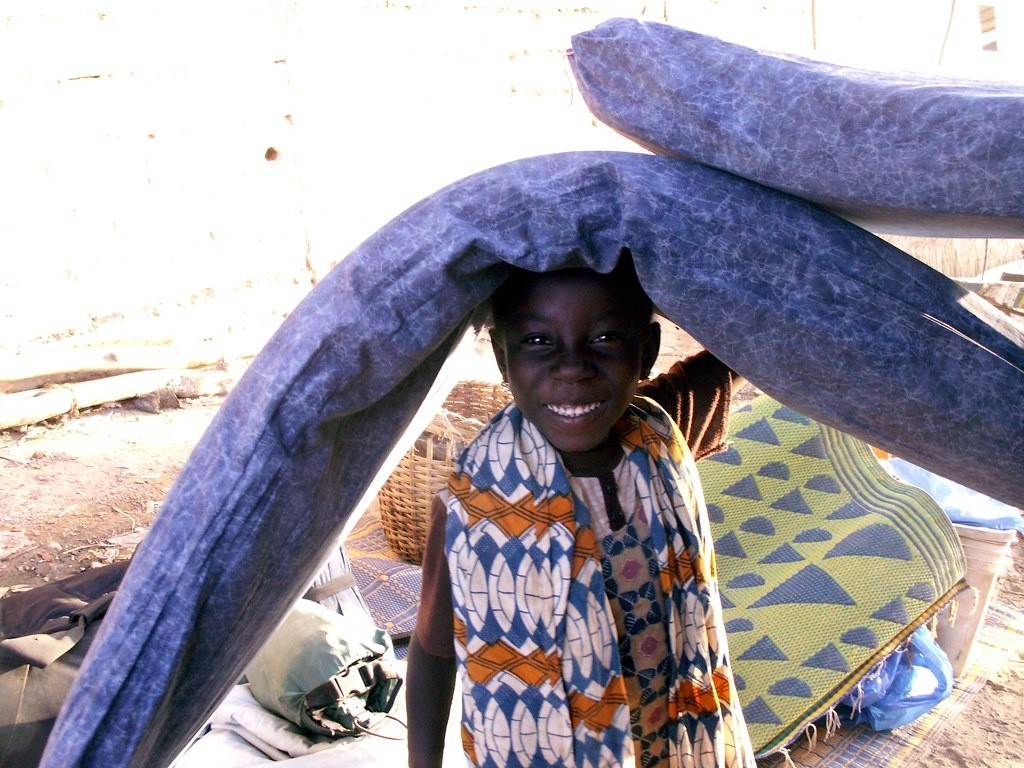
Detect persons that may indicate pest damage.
[406,258,756,768]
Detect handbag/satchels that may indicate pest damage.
[244,598,409,741]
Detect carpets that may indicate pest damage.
[693,392,970,755]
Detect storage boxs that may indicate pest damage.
[928,528,1016,684]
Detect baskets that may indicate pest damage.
[377,378,514,563]
